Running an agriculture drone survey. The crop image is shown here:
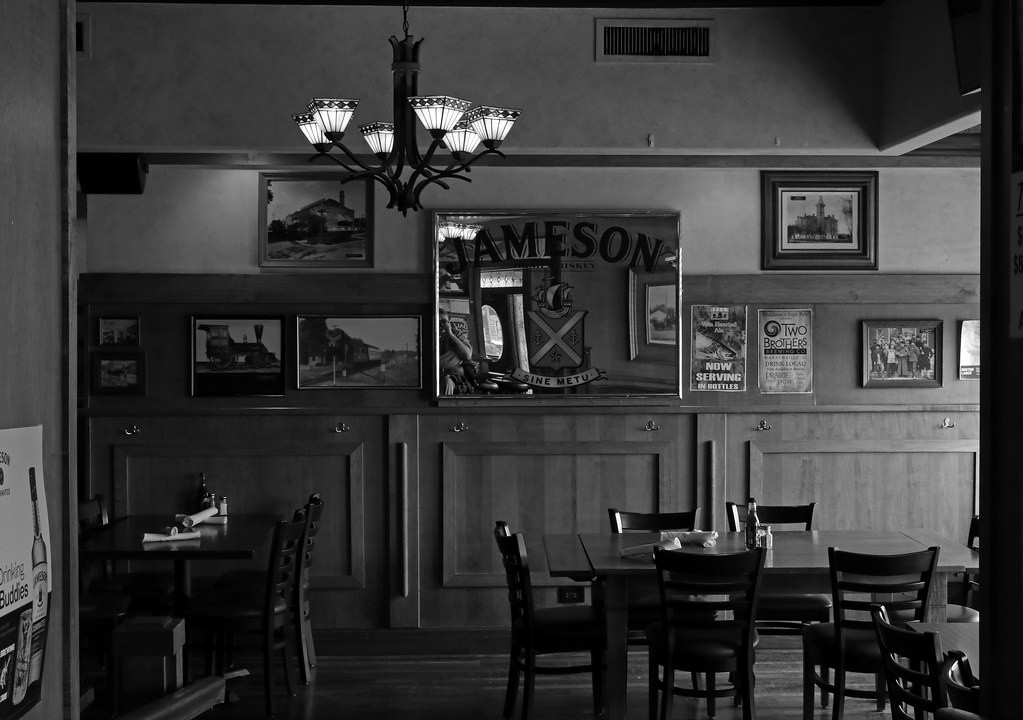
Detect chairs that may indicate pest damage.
[74,492,324,720]
[496,501,982,720]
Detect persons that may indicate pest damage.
[870,331,934,380]
[439,307,473,396]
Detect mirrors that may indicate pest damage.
[628,262,678,366]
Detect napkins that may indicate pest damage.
[692,528,716,548]
[660,531,719,543]
[185,506,219,528]
[160,525,179,536]
[175,513,228,526]
[137,529,201,542]
[619,529,682,558]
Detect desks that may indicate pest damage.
[906,623,982,720]
[79,513,283,707]
[541,527,982,720]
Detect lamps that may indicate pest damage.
[434,221,484,260]
[290,0,522,219]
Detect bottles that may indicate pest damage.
[27,466,50,693]
[197,473,210,511]
[210,493,216,508]
[745,498,767,551]
[218,496,227,516]
[756,526,773,550]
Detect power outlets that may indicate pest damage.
[558,586,585,604]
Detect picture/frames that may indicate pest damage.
[643,281,678,349]
[426,207,685,404]
[98,313,141,348]
[91,350,149,398]
[759,168,880,272]
[257,171,376,269]
[188,312,286,398]
[858,318,944,388]
[296,313,423,390]
